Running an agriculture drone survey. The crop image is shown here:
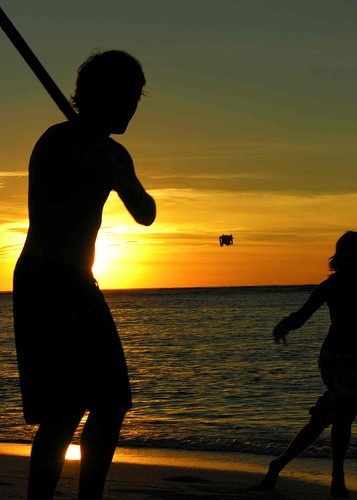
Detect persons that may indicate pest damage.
[258,229,357,500]
[12,49,157,500]
